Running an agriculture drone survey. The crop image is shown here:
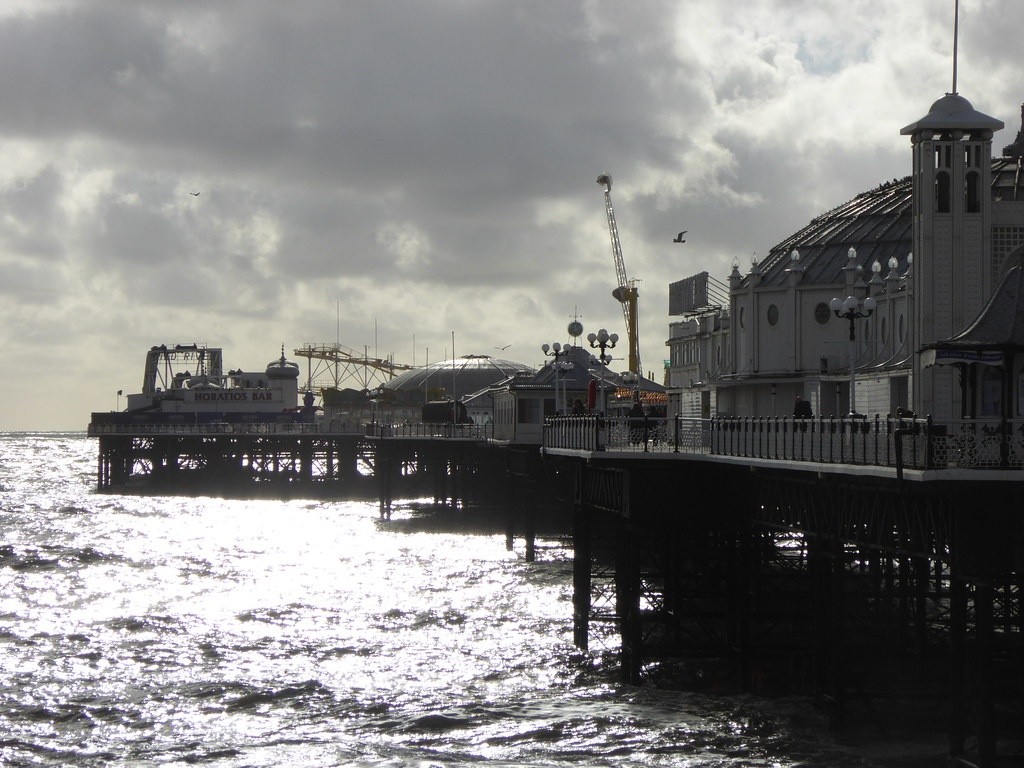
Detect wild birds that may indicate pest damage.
[189,192,200,197]
[672,230,688,243]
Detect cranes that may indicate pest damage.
[595,171,645,377]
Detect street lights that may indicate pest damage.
[830,295,877,418]
[542,342,572,412]
[552,361,575,416]
[587,328,619,417]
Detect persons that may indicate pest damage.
[794,396,815,432]
[572,399,662,446]
